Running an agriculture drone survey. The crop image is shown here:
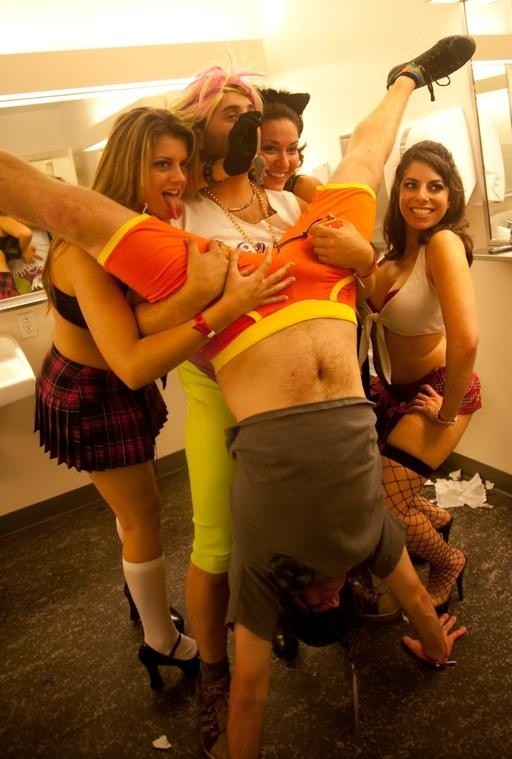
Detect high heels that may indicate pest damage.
[124,583,184,629]
[425,553,467,614]
[138,632,199,688]
[409,517,454,564]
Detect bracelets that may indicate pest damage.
[355,241,378,279]
[435,409,459,426]
[420,638,456,668]
[192,311,216,341]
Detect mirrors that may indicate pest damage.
[1,75,225,310]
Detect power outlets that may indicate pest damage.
[18,312,38,340]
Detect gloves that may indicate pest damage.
[223,110,261,175]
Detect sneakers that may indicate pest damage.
[352,579,399,621]
[387,36,475,100]
[199,685,231,759]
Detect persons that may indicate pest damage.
[368,139,482,617]
[184,86,325,665]
[162,64,407,759]
[2,210,43,300]
[32,105,199,693]
[2,36,476,757]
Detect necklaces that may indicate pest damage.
[226,189,257,216]
[206,180,276,254]
